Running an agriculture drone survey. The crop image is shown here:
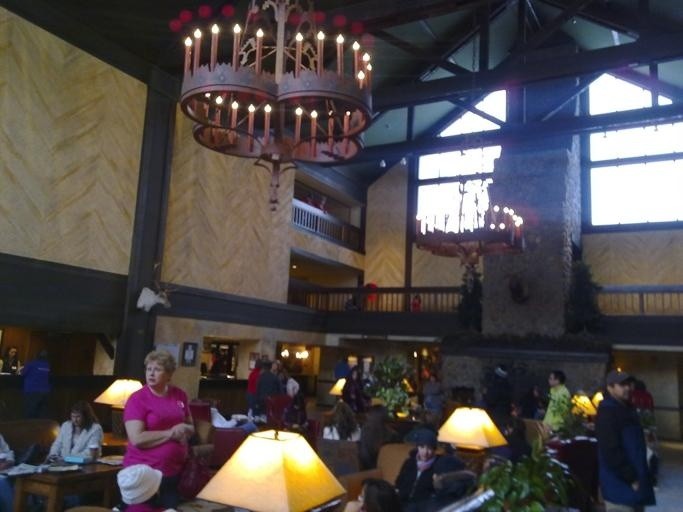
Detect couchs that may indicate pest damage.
[266,394,320,444]
[336,444,447,511]
[210,426,244,469]
[189,402,212,426]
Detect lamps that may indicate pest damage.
[177,0,373,207]
[590,391,603,407]
[414,142,525,286]
[435,407,512,473]
[197,427,346,511]
[566,389,598,421]
[93,379,144,436]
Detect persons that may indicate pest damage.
[122,349,194,508]
[210,351,223,377]
[393,428,443,502]
[0,344,20,374]
[217,405,258,435]
[629,380,654,413]
[43,399,104,463]
[401,453,479,511]
[354,476,401,511]
[112,463,177,511]
[316,355,572,468]
[247,357,307,425]
[18,348,50,421]
[595,368,655,511]
[0,432,21,512]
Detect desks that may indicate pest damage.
[12,464,124,512]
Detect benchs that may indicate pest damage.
[0,416,62,467]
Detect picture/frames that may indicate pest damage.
[179,340,199,370]
[247,352,261,370]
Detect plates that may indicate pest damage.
[104,455,124,462]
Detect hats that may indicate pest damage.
[606,370,634,385]
[117,464,162,504]
[415,429,436,445]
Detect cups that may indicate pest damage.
[88,445,99,461]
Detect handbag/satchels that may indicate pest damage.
[176,456,212,498]
